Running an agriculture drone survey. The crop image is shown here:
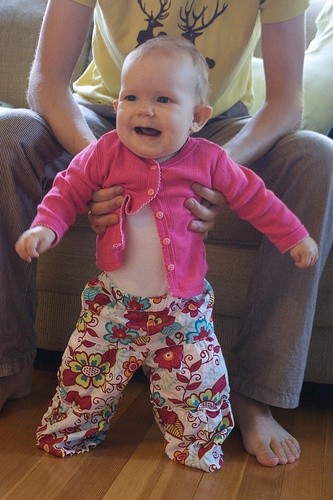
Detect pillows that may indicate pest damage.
[248,1,333,135]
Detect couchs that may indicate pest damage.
[0,1,333,391]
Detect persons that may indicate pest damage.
[0,0,333,467]
[14,36,319,473]
